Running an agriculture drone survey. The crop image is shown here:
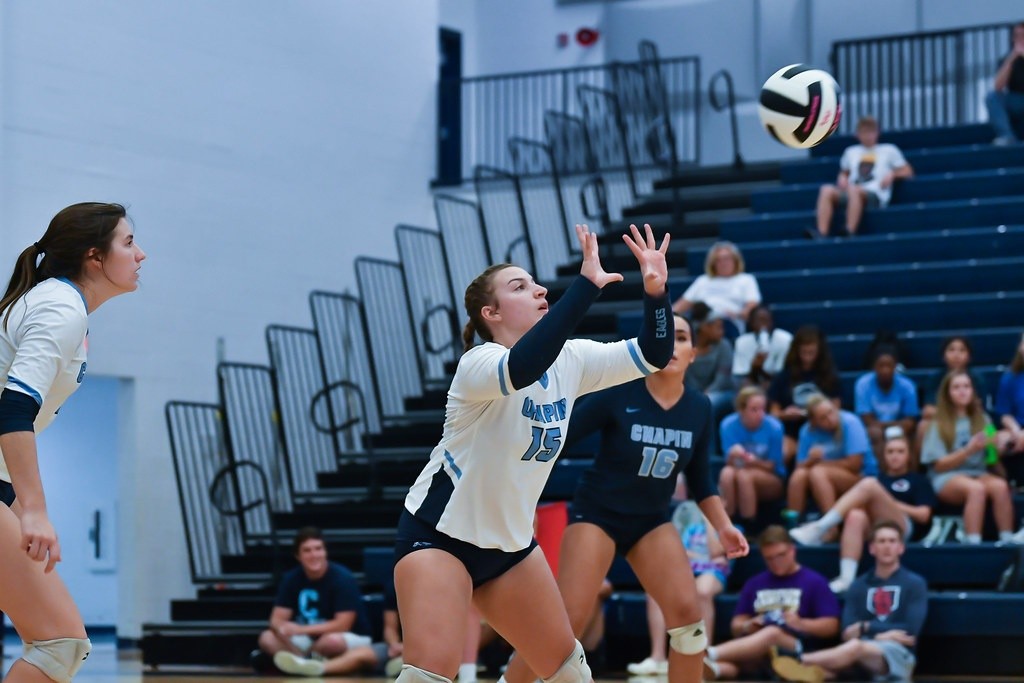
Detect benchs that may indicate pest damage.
[137,141,1024,674]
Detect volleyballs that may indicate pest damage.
[759,62,842,150]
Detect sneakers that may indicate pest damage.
[627,656,668,673]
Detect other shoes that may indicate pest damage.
[789,522,824,546]
[772,656,826,683]
[828,576,851,593]
[384,656,404,678]
[275,652,324,676]
[250,650,271,673]
[770,645,802,663]
[701,648,719,680]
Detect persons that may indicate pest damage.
[674,240,762,338]
[798,117,912,242]
[985,23,1024,145]
[253,300,1024,683]
[0,203,145,683]
[393,223,675,683]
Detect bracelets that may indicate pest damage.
[860,622,864,633]
